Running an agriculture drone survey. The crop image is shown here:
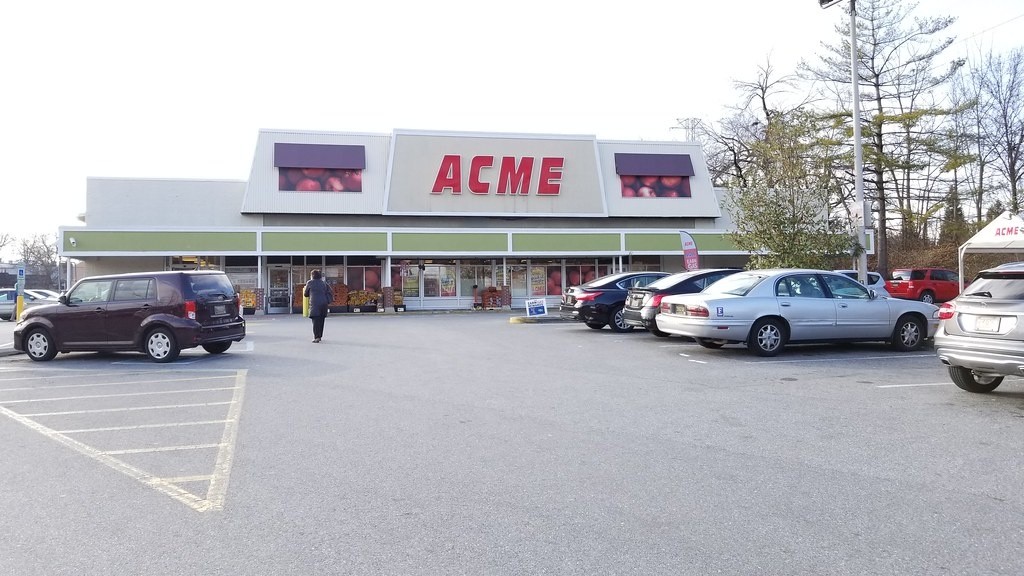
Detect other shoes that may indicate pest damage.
[312,337,321,343]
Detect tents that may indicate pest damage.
[958,212,1024,294]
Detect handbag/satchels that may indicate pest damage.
[324,282,333,304]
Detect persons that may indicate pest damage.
[9,283,18,322]
[304,268,334,343]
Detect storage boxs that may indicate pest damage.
[473,286,511,310]
[382,287,395,308]
[253,288,266,310]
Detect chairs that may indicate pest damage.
[117,284,136,299]
[796,283,822,297]
[778,284,789,295]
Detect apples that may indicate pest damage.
[546,265,601,295]
[277,167,363,192]
[351,270,380,293]
[620,174,690,198]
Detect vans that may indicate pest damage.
[821,270,892,297]
[884,268,970,304]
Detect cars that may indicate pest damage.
[622,268,744,337]
[560,271,672,332]
[655,269,942,357]
[0,288,58,320]
[0,289,60,301]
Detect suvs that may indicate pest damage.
[933,261,1024,393]
[14,269,246,363]
